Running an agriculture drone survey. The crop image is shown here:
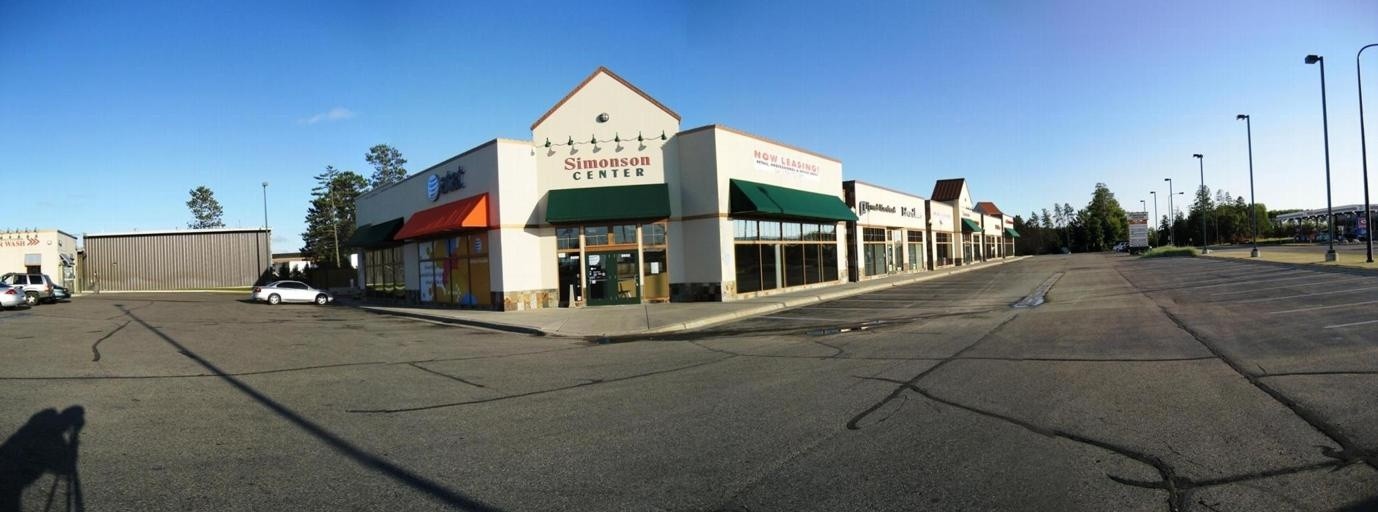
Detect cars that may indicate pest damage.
[52,285,70,301]
[1110,240,1129,252]
[251,279,336,307]
[0,282,26,310]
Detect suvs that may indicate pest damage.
[1,273,54,307]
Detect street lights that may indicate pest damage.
[1150,191,1159,248]
[1303,52,1339,263]
[1164,178,1176,246]
[1193,154,1209,254]
[1235,113,1261,260]
[1168,192,1185,246]
[262,180,273,276]
[1140,200,1147,212]
[324,163,342,269]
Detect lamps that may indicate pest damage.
[541,130,668,151]
